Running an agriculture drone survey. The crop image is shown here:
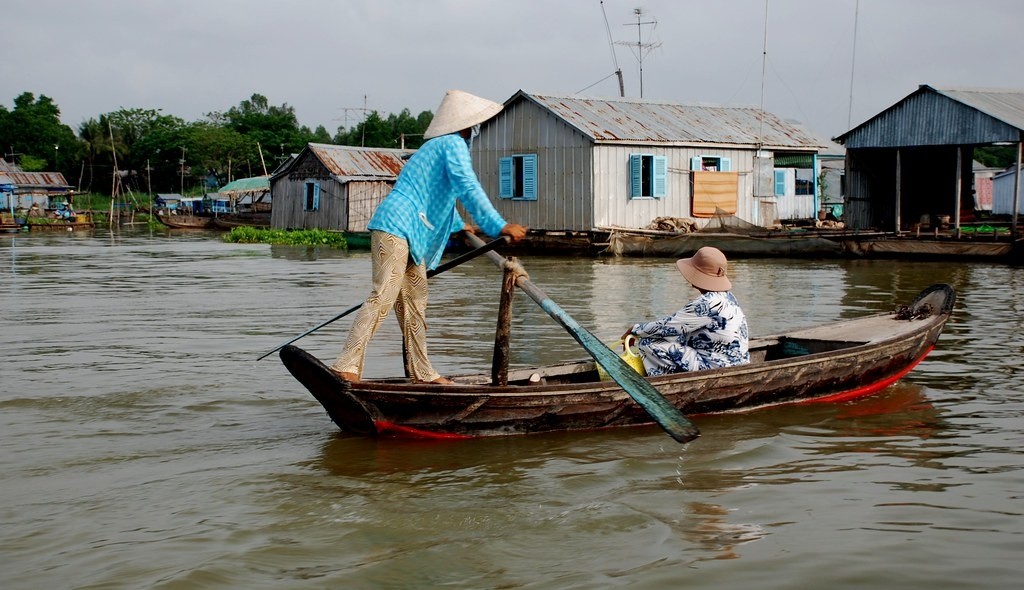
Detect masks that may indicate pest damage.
[467,124,481,154]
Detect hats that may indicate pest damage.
[677,247,732,292]
[423,89,504,139]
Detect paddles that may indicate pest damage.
[255,232,508,361]
[475,228,705,442]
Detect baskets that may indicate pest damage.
[595,334,645,382]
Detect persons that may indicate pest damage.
[326,89,531,385]
[619,245,752,377]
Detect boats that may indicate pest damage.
[211,209,272,231]
[279,279,957,436]
[143,205,220,229]
